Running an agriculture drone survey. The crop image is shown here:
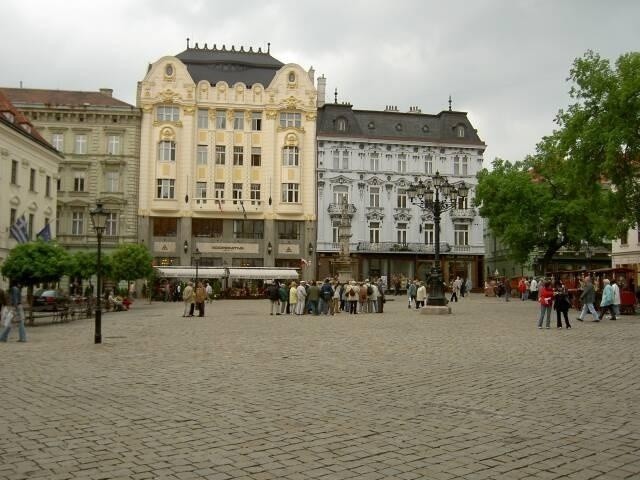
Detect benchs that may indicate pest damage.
[11,296,114,326]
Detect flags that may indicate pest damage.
[38,223,50,244]
[10,213,30,245]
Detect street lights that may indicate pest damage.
[88,202,109,344]
[407,170,470,306]
[192,247,202,310]
[221,261,229,298]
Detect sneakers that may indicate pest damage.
[538,326,571,330]
[609,318,616,320]
[0,337,26,342]
[576,317,583,322]
[182,314,204,317]
[592,320,600,322]
[270,308,383,316]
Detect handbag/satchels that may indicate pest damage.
[345,292,349,297]
[4,312,21,328]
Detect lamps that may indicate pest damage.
[184,241,188,253]
[309,243,314,254]
[268,242,272,254]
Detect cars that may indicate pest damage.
[30,289,70,312]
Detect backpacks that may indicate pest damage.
[367,287,373,296]
[350,289,355,296]
[322,286,332,300]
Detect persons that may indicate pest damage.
[1,281,32,342]
[65,276,136,321]
[147,267,638,331]
